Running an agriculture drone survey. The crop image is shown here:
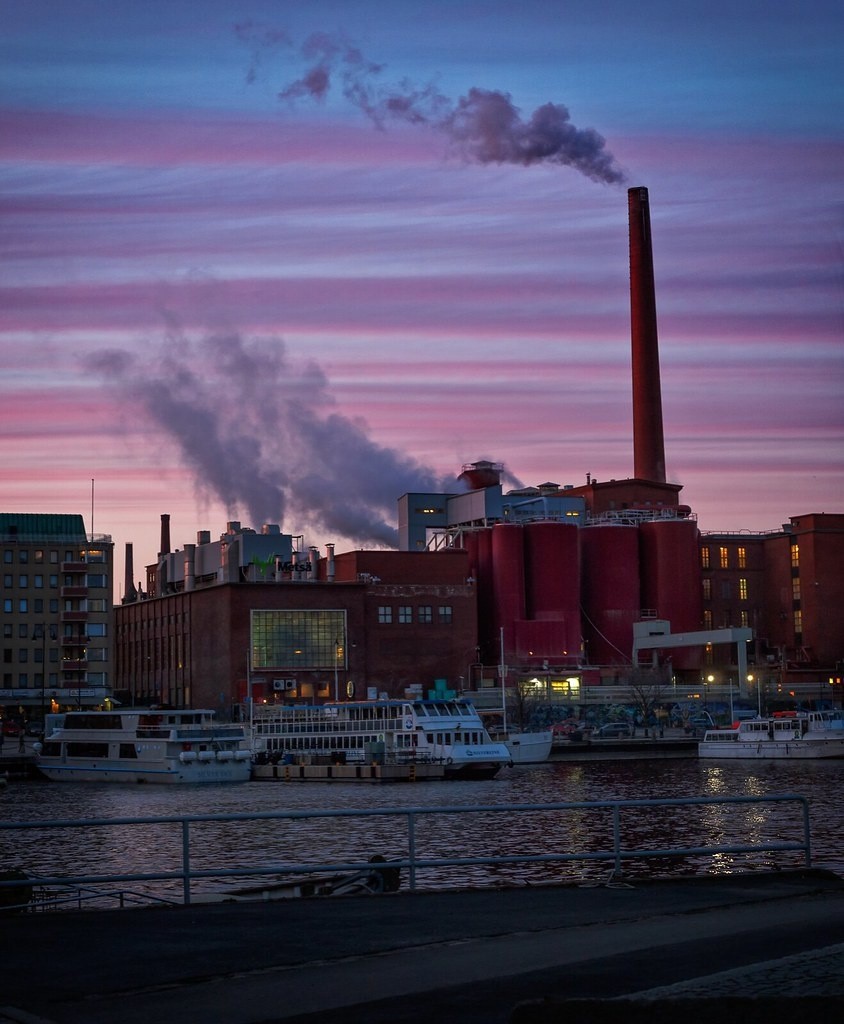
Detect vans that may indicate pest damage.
[597,723,629,737]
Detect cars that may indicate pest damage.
[0,721,26,737]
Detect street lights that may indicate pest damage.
[31,621,58,714]
[828,677,841,712]
[702,674,714,711]
[77,624,92,710]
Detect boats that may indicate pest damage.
[160,856,407,905]
[699,717,844,759]
[32,709,255,784]
[320,699,515,782]
